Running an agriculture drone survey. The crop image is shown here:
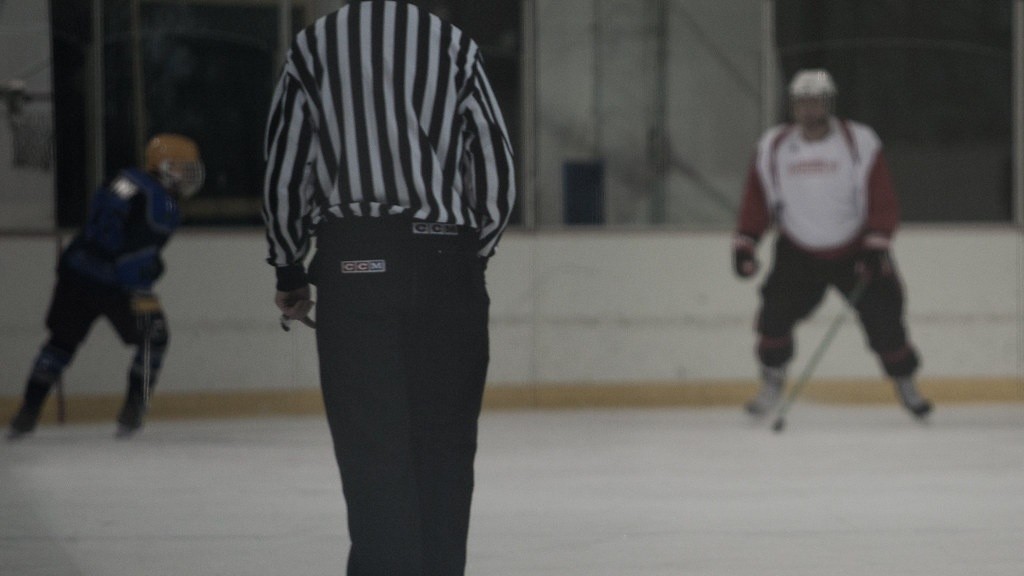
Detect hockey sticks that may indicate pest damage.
[766,268,872,431]
[55,213,67,423]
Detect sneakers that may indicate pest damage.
[892,377,932,420]
[7,412,38,439]
[740,363,787,427]
[115,405,143,437]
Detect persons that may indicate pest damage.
[260,0,518,576]
[7,132,205,442]
[732,67,935,426]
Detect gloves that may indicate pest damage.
[849,248,889,299]
[735,246,755,277]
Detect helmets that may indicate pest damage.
[147,135,197,170]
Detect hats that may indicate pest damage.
[790,67,836,96]
